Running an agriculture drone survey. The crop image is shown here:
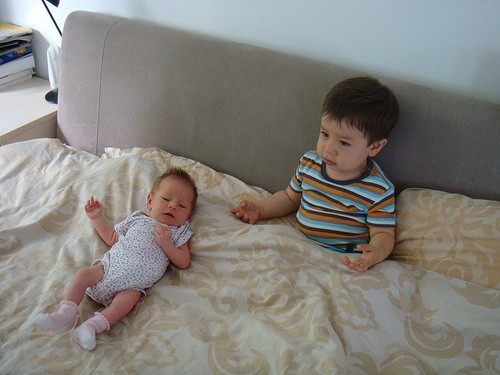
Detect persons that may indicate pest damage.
[35,167,198,352]
[230,76,400,274]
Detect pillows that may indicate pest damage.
[388,187,500,291]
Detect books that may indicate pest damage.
[0,21,37,89]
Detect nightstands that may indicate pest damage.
[0,76,58,146]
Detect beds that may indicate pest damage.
[0,11,500,375]
